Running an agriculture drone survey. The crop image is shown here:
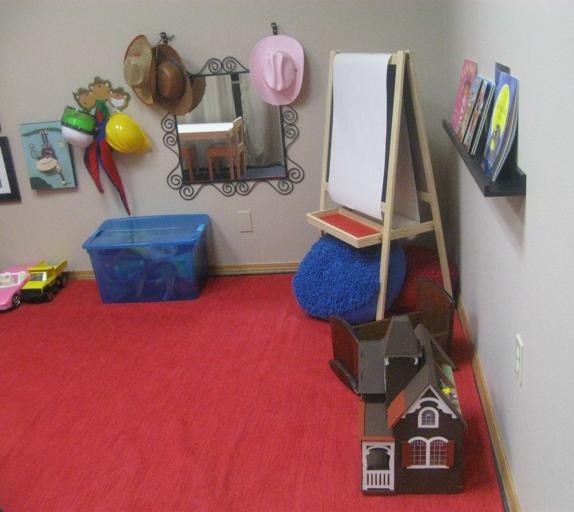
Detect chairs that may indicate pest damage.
[179,144,200,180]
[208,116,246,178]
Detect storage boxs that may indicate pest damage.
[81,213,212,305]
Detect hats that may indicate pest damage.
[149,42,207,118]
[121,34,159,107]
[247,33,306,107]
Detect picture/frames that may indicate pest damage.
[0,136,21,202]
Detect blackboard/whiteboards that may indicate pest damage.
[321,52,407,213]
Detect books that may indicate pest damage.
[449,58,520,183]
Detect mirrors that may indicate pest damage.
[160,57,305,200]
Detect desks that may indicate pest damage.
[176,123,244,180]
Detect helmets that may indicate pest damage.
[59,104,96,149]
[103,111,156,158]
